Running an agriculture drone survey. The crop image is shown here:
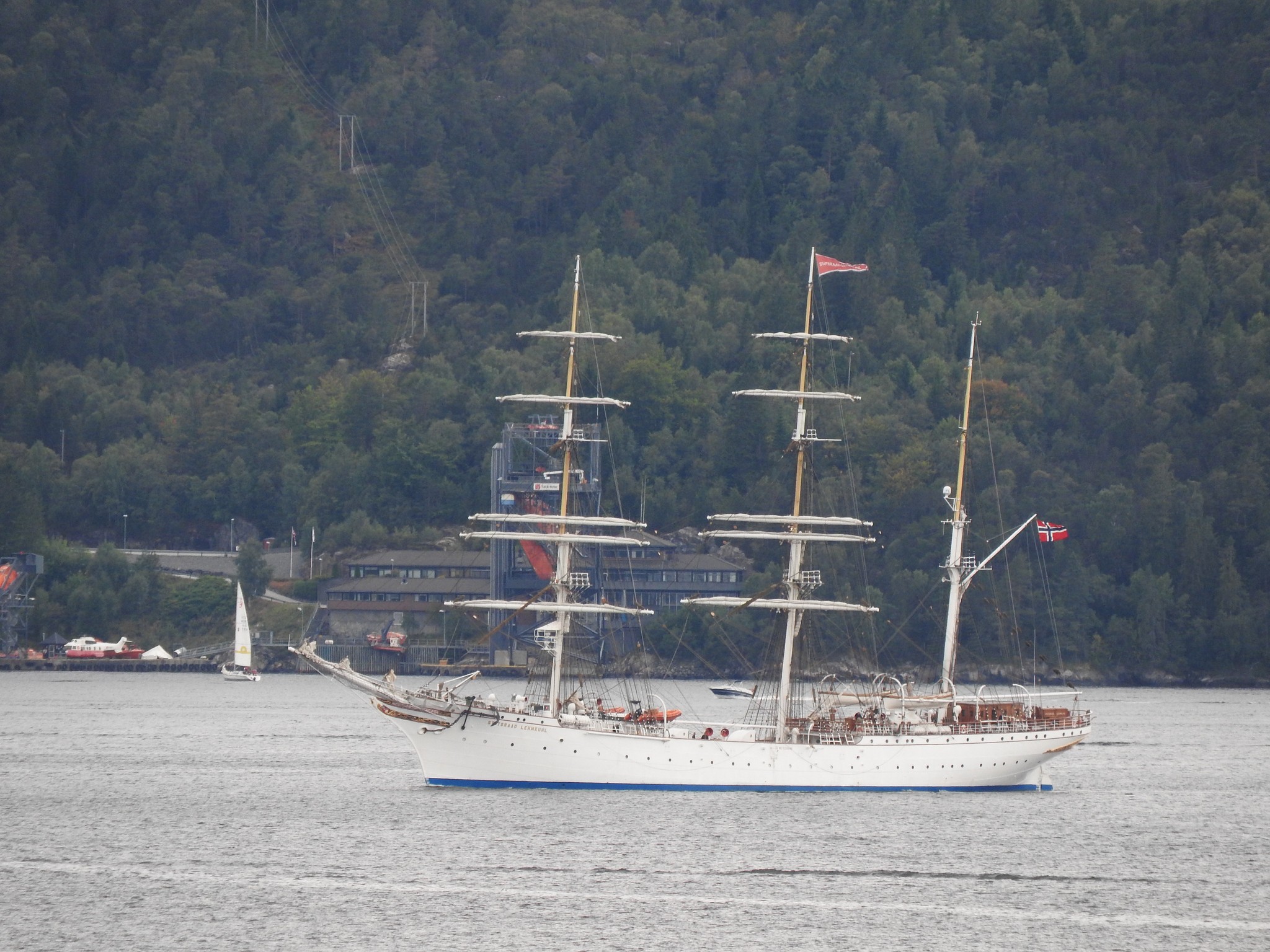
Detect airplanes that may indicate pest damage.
[63,634,145,659]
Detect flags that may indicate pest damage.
[1035,518,1069,542]
[815,253,869,276]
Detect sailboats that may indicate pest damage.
[221,579,261,682]
[284,246,1096,793]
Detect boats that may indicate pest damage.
[708,679,753,698]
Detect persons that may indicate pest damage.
[692,732,695,739]
[873,706,879,715]
[445,687,449,701]
[702,732,708,739]
[244,667,249,671]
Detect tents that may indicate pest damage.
[141,645,173,660]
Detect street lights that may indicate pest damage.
[123,515,128,549]
[230,518,234,552]
